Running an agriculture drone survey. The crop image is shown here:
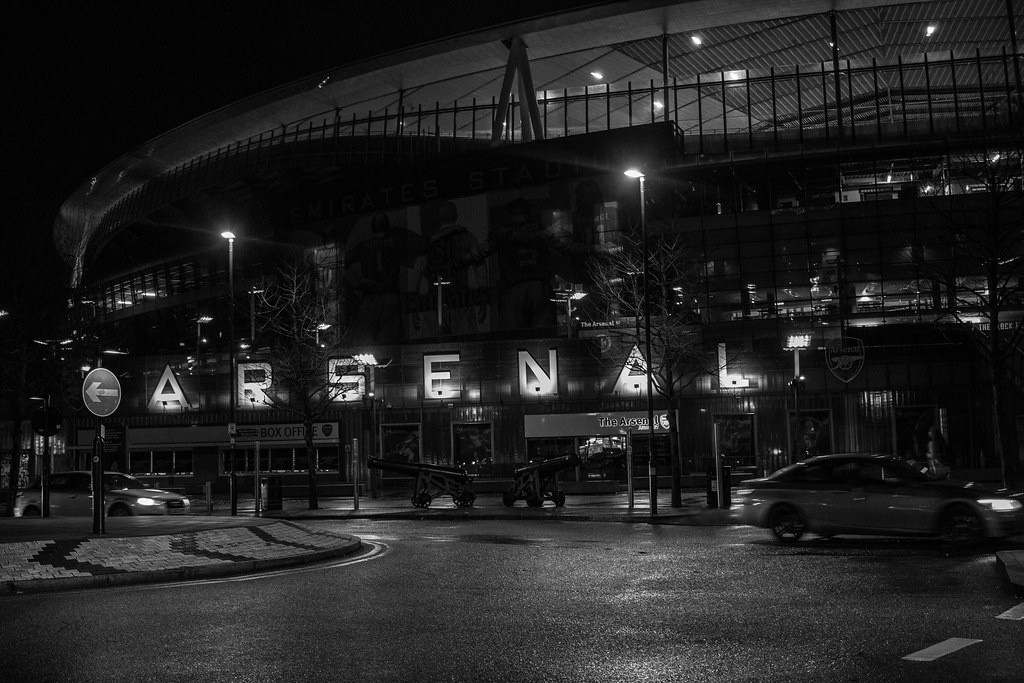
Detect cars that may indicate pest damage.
[12,469,192,518]
[738,454,1024,550]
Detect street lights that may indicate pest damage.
[566,291,590,337]
[316,323,332,346]
[96,346,130,535]
[32,336,75,517]
[194,316,214,374]
[221,228,241,517]
[622,164,661,517]
[433,282,452,338]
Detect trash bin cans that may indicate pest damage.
[706,465,732,507]
[261,476,283,510]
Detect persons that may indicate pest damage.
[927,428,947,468]
[345,214,415,342]
[482,177,590,330]
[465,428,490,457]
[110,462,119,479]
[401,434,419,462]
[413,201,487,333]
